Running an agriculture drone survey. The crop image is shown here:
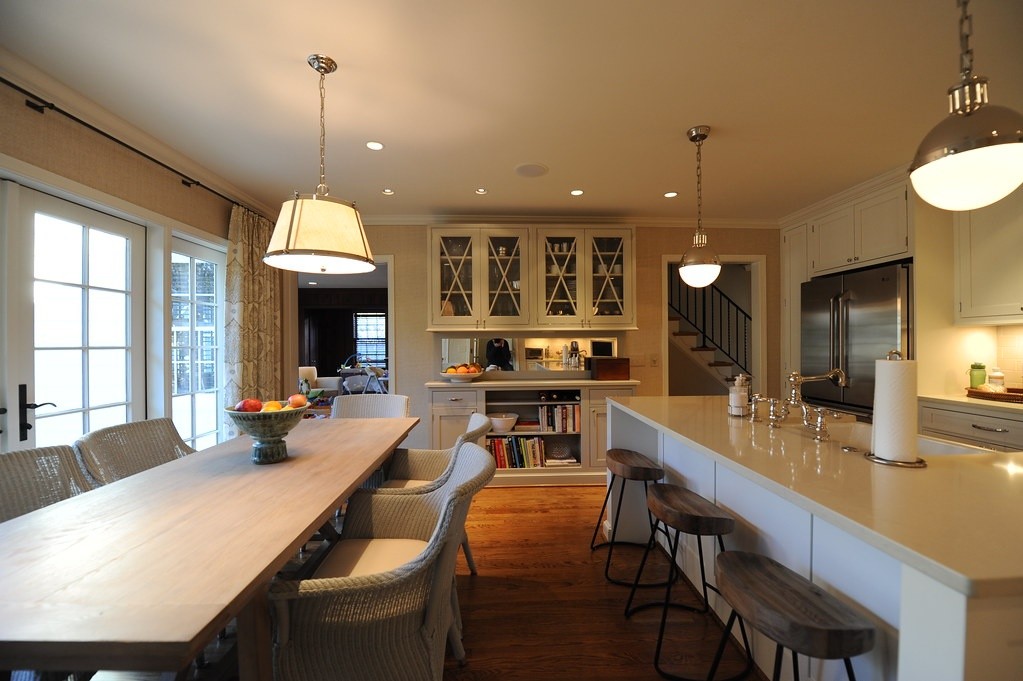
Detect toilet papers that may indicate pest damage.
[870,359,918,462]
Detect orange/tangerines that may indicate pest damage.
[447,366,467,373]
[260,401,294,412]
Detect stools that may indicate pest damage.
[711,549,874,680]
[589,448,677,588]
[622,484,737,680]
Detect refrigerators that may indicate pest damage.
[796,264,914,425]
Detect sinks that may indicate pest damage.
[788,425,997,455]
[748,391,856,423]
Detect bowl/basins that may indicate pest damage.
[224,401,312,464]
[486,412,519,433]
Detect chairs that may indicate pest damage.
[1,443,91,523]
[74,416,195,487]
[331,395,408,420]
[350,406,491,626]
[239,442,496,681]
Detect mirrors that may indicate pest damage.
[442,337,617,372]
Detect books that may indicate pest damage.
[539,405,581,433]
[545,455,581,468]
[486,436,545,468]
[515,421,540,431]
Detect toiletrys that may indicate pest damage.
[728,374,749,416]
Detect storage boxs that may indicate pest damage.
[591,356,630,380]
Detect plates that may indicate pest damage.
[439,372,483,382]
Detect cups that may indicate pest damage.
[551,264,567,273]
[512,280,520,290]
[599,264,622,274]
[593,307,598,315]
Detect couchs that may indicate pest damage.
[298,367,343,398]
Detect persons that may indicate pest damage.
[485,338,513,371]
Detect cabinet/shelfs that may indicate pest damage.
[428,222,641,332]
[427,386,635,485]
[954,183,1023,326]
[783,224,807,398]
[807,162,913,279]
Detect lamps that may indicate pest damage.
[679,124,723,288]
[910,0,1023,210]
[262,52,375,275]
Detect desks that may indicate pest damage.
[598,396,1023,681]
[0,415,419,681]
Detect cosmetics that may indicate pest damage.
[561,343,580,368]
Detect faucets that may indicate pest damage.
[748,368,847,443]
[795,401,842,442]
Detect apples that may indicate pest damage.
[288,394,306,407]
[467,366,478,373]
[234,399,262,412]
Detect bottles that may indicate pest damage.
[548,309,570,315]
[988,368,1005,388]
[969,362,986,389]
[562,344,580,369]
